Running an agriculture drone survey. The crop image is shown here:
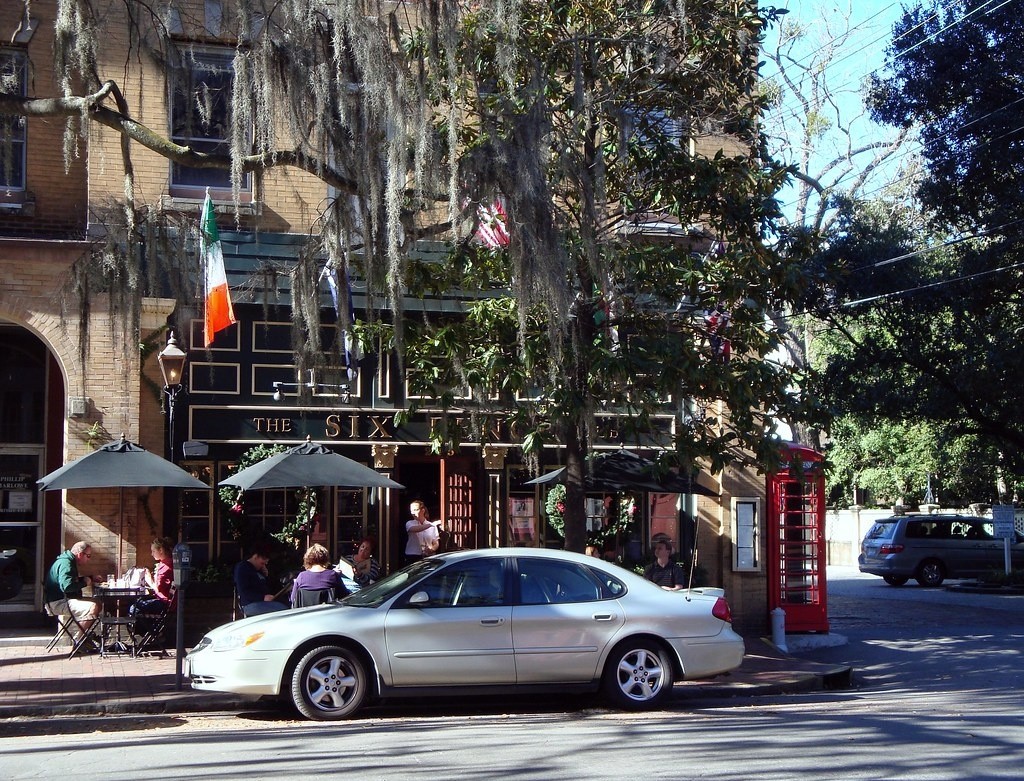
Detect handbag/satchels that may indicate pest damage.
[123,566,146,594]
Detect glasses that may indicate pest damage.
[84,553,91,558]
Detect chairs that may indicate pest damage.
[296,587,338,610]
[953,525,962,538]
[96,589,142,659]
[232,583,248,624]
[39,576,101,660]
[459,575,488,605]
[135,581,180,657]
[917,526,929,538]
[964,527,978,540]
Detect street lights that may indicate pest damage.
[156,329,188,695]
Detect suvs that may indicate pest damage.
[858,514,1024,587]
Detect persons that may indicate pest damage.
[129,537,177,632]
[42,542,103,647]
[290,544,335,608]
[334,542,379,593]
[234,549,287,615]
[404,499,440,561]
[642,540,683,591]
[586,546,599,559]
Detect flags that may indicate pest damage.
[199,197,236,347]
[571,287,619,354]
[460,179,510,248]
[706,245,732,365]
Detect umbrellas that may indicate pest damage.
[37,434,211,577]
[521,449,722,498]
[217,434,406,547]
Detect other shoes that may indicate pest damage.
[78,647,87,653]
[87,641,100,647]
[143,637,166,646]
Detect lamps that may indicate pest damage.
[272,381,287,404]
[340,385,350,405]
[11,11,40,48]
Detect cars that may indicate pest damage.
[185,552,746,721]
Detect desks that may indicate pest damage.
[91,583,153,655]
